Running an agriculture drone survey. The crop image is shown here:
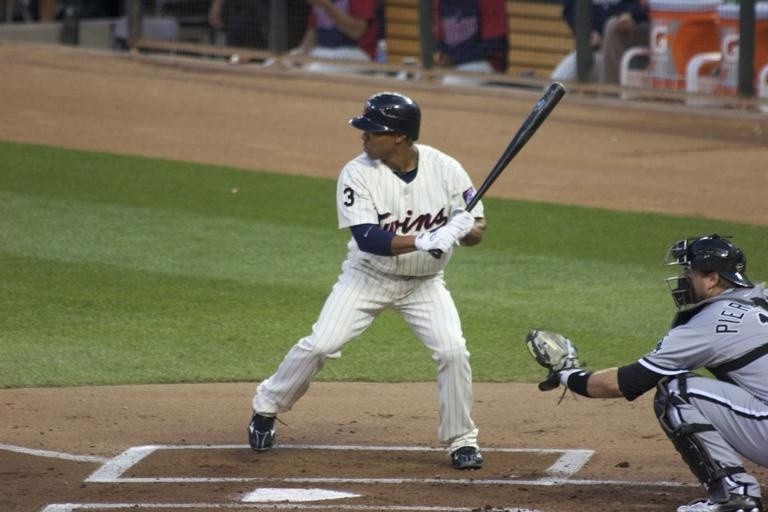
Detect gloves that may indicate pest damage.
[450,211,475,242]
[415,225,459,255]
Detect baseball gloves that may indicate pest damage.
[526,329,582,408]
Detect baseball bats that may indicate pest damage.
[429,82,566,259]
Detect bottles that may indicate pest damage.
[376,38,388,79]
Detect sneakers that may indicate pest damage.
[451,446,484,469]
[678,493,764,512]
[246,410,279,452]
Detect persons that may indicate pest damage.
[263,0,381,78]
[204,1,311,68]
[551,0,652,96]
[247,89,488,471]
[524,233,767,512]
[430,0,510,90]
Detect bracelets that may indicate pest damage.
[566,369,592,400]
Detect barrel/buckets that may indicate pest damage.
[649,0,768,99]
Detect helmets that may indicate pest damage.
[348,91,423,139]
[662,234,756,310]
